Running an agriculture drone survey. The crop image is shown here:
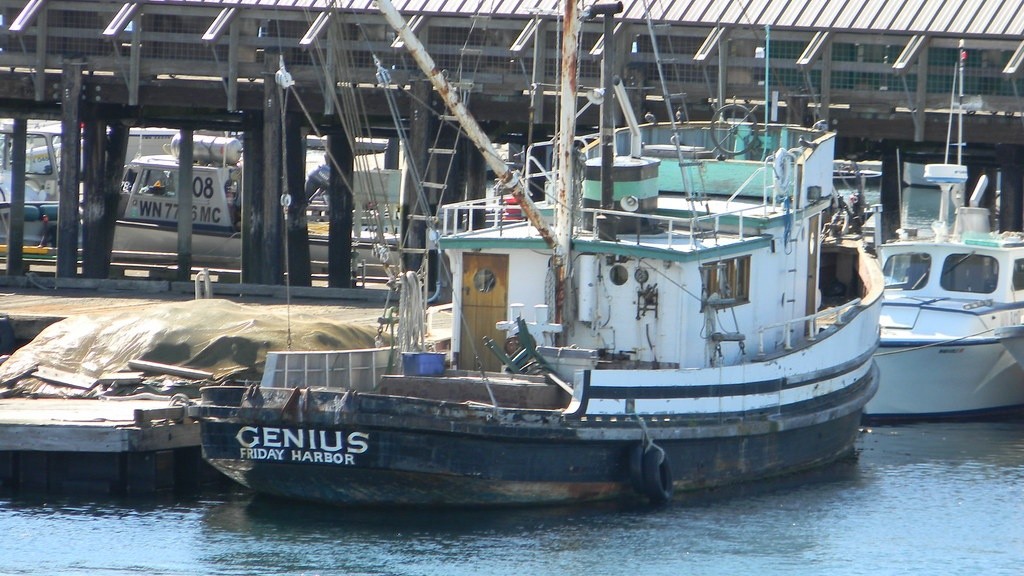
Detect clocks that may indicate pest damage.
[634,270,649,282]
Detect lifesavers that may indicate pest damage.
[643,442,673,508]
[626,445,644,494]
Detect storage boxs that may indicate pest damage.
[402,352,447,376]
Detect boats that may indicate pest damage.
[2,2,1024,520]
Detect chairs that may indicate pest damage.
[23,205,40,246]
[40,205,59,247]
